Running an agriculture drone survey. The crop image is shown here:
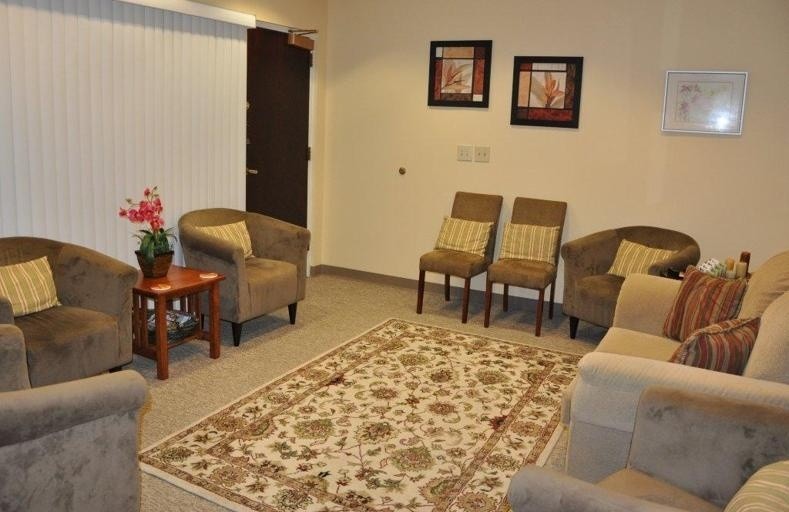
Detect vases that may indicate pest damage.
[134,249,175,278]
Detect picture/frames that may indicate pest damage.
[660,69,749,136]
[509,54,584,128]
[426,39,492,108]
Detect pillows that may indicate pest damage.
[195,220,254,261]
[433,215,493,257]
[724,460,788,511]
[607,238,679,279]
[498,221,561,267]
[669,316,762,377]
[0,256,62,318]
[662,265,747,343]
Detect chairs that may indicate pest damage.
[1,324,151,512]
[416,190,504,323]
[508,386,788,512]
[484,194,568,338]
[0,235,139,392]
[176,207,312,348]
[560,225,700,341]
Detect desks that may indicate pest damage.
[127,265,226,380]
[661,266,752,281]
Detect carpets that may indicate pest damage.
[139,316,584,512]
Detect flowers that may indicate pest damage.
[117,185,178,264]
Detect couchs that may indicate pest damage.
[557,251,789,487]
